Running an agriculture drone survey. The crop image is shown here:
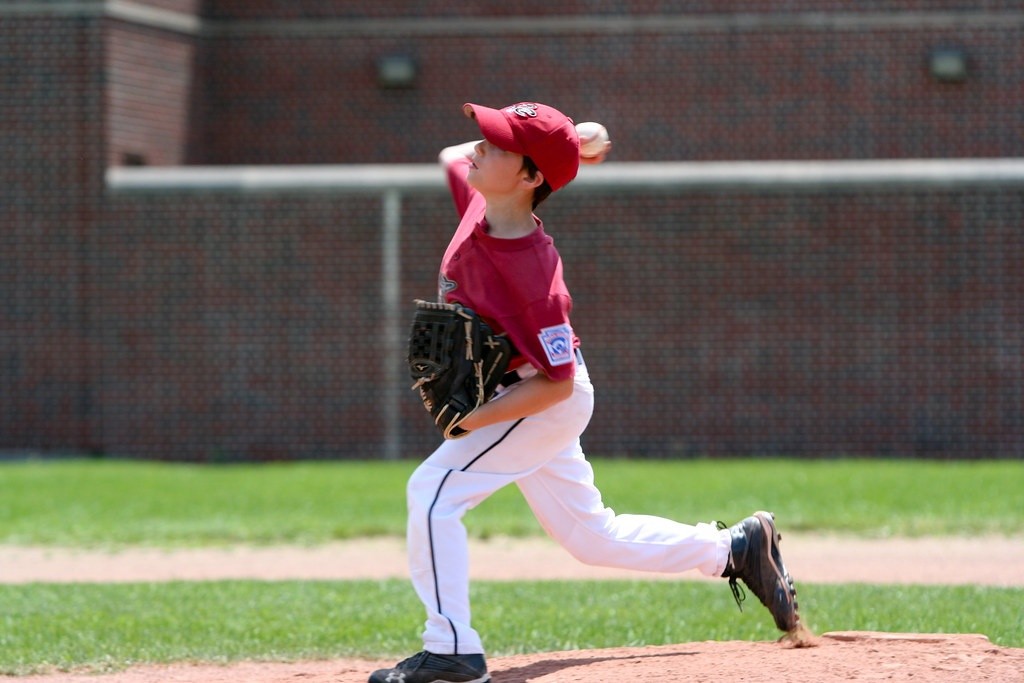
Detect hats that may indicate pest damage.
[463,100,580,193]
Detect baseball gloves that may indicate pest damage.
[406,295,512,439]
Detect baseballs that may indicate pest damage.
[575,120,608,158]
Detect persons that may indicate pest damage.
[368,100,799,681]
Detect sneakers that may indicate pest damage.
[717,510,799,633]
[368,650,492,683]
